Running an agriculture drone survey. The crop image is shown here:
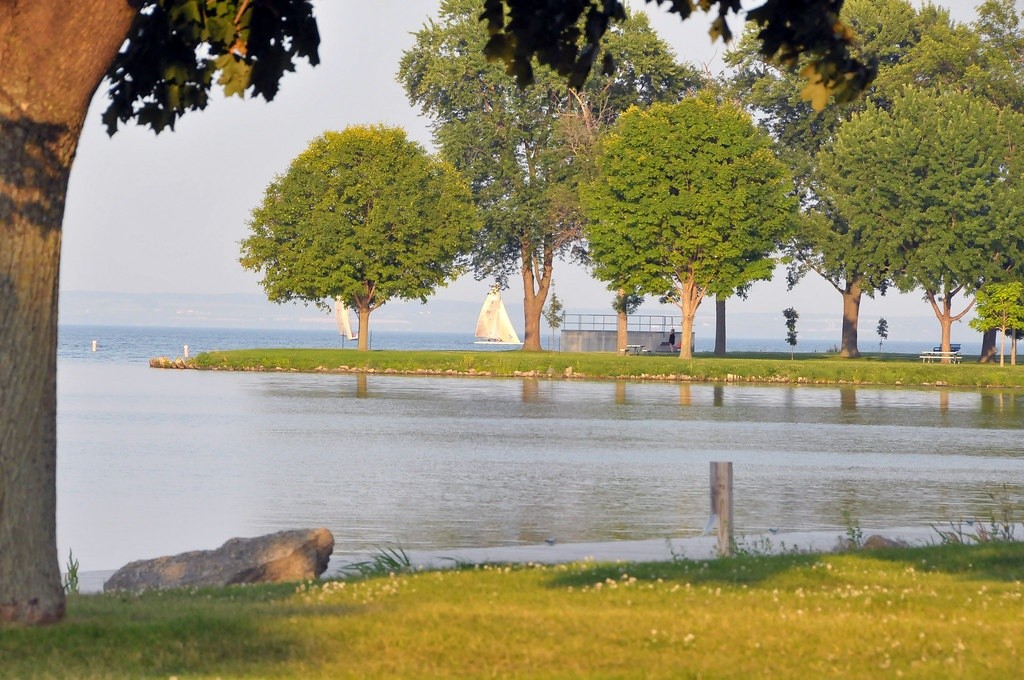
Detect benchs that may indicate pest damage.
[920,355,963,365]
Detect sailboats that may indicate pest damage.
[334,293,359,341]
[473,281,525,346]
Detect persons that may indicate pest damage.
[668,329,675,354]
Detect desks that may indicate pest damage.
[922,351,958,364]
[627,345,644,356]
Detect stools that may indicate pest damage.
[620,348,630,356]
[642,349,651,353]
